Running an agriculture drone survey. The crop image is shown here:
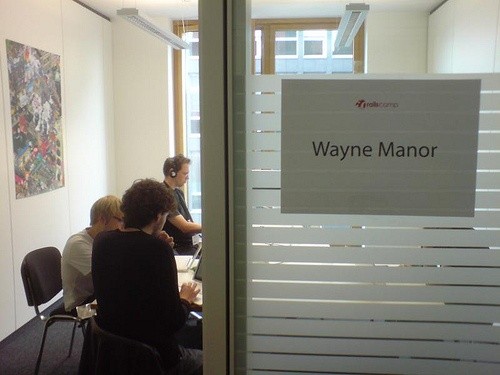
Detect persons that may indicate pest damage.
[90,178,203,375]
[60,194,124,318]
[159,153,202,256]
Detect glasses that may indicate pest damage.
[112,216,124,225]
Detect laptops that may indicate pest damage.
[173,242,202,272]
[177,256,203,308]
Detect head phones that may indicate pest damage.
[170,158,177,177]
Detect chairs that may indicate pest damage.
[78,317,164,375]
[22,247,89,375]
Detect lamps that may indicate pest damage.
[114,0,189,51]
[334,0,370,49]
